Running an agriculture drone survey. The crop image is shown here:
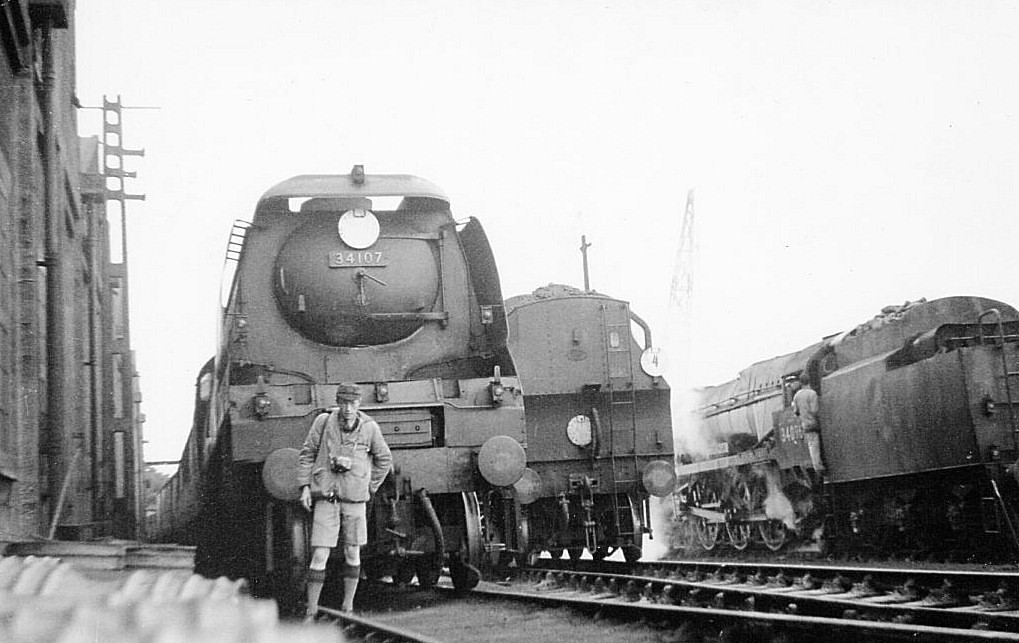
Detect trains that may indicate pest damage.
[145,163,680,596]
[678,293,1019,562]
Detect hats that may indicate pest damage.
[337,382,362,401]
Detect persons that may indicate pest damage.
[298,382,392,624]
[791,372,828,473]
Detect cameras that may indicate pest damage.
[332,455,352,472]
